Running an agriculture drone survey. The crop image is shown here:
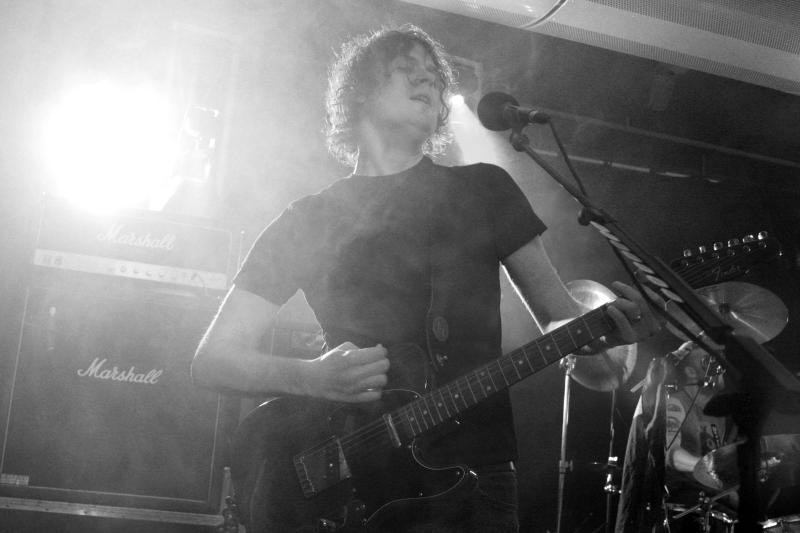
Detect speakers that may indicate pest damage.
[0,291,234,513]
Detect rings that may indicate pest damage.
[633,310,644,322]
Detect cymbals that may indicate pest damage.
[558,279,637,392]
[655,434,800,533]
[663,281,790,350]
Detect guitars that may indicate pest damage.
[230,232,784,533]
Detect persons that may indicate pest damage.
[189,23,669,533]
[663,347,800,533]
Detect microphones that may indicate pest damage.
[478,91,550,132]
